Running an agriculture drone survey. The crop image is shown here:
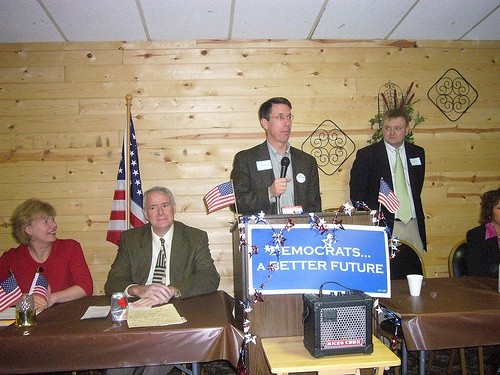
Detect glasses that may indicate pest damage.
[268,114,294,121]
[148,204,169,211]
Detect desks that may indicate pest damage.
[261,336,401,375]
[372,276,500,375]
[0,290,244,375]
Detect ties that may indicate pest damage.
[394,148,413,224]
[152,238,168,287]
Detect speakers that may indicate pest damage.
[303,292,373,358]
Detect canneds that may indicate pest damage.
[111,292,128,322]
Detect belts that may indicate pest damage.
[395,217,413,222]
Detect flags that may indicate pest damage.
[106,112,145,244]
[204,181,235,213]
[378,179,400,213]
[0,275,23,313]
[29,273,48,301]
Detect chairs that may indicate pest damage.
[376,239,484,375]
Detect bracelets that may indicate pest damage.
[174,292,181,298]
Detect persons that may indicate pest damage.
[349,109,427,279]
[0,198,93,311]
[233,97,321,215]
[465,188,500,369]
[104,187,220,375]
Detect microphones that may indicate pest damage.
[280,157,290,178]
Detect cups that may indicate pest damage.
[407,274,423,297]
[15,293,37,325]
